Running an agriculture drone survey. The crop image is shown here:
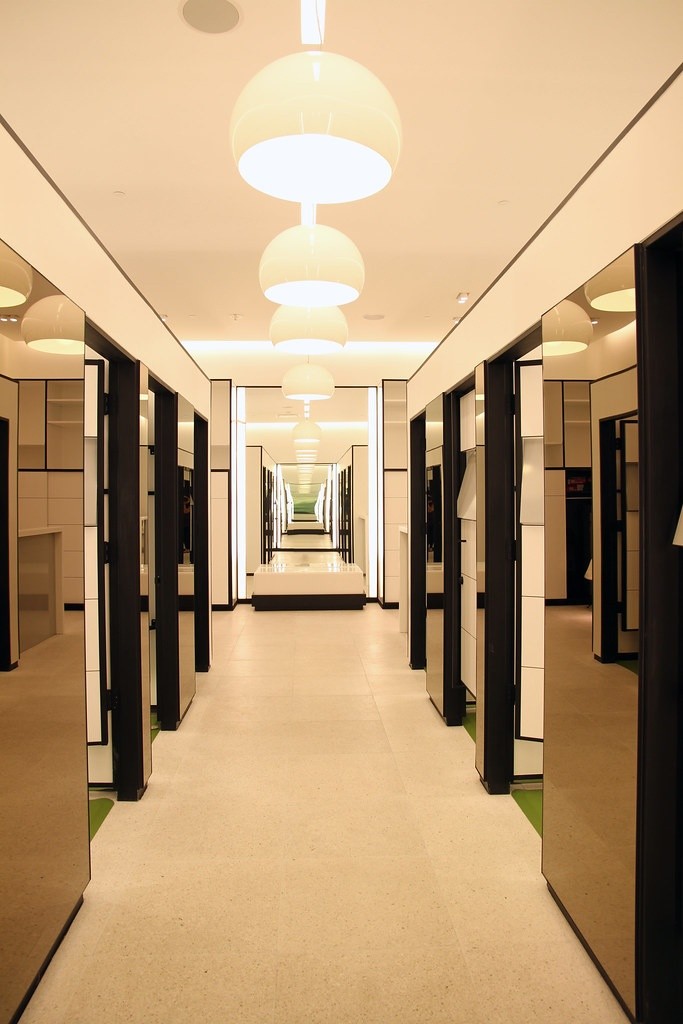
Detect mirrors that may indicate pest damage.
[211,379,234,611]
[176,395,199,721]
[275,462,339,552]
[381,378,410,611]
[473,362,486,780]
[538,244,648,1024]
[236,385,379,602]
[424,392,446,717]
[138,360,153,789]
[0,241,95,1024]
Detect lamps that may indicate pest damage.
[541,299,595,359]
[20,292,85,356]
[0,241,34,309]
[268,304,348,357]
[259,223,367,309]
[290,419,322,463]
[584,246,642,312]
[281,363,335,401]
[228,49,404,207]
